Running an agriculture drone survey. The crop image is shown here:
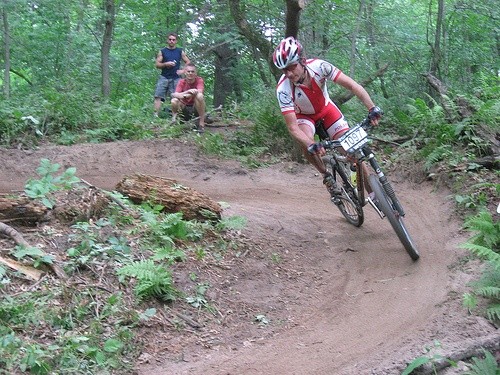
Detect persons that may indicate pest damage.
[273,36,384,194]
[153,33,190,117]
[171,63,206,130]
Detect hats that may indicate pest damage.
[272,35,302,69]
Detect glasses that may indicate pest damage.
[281,58,300,70]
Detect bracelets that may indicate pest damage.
[184,94,186,98]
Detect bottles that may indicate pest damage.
[349,162,357,187]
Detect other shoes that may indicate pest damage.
[321,173,341,194]
[346,151,357,171]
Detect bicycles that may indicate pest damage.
[305,109,420,262]
